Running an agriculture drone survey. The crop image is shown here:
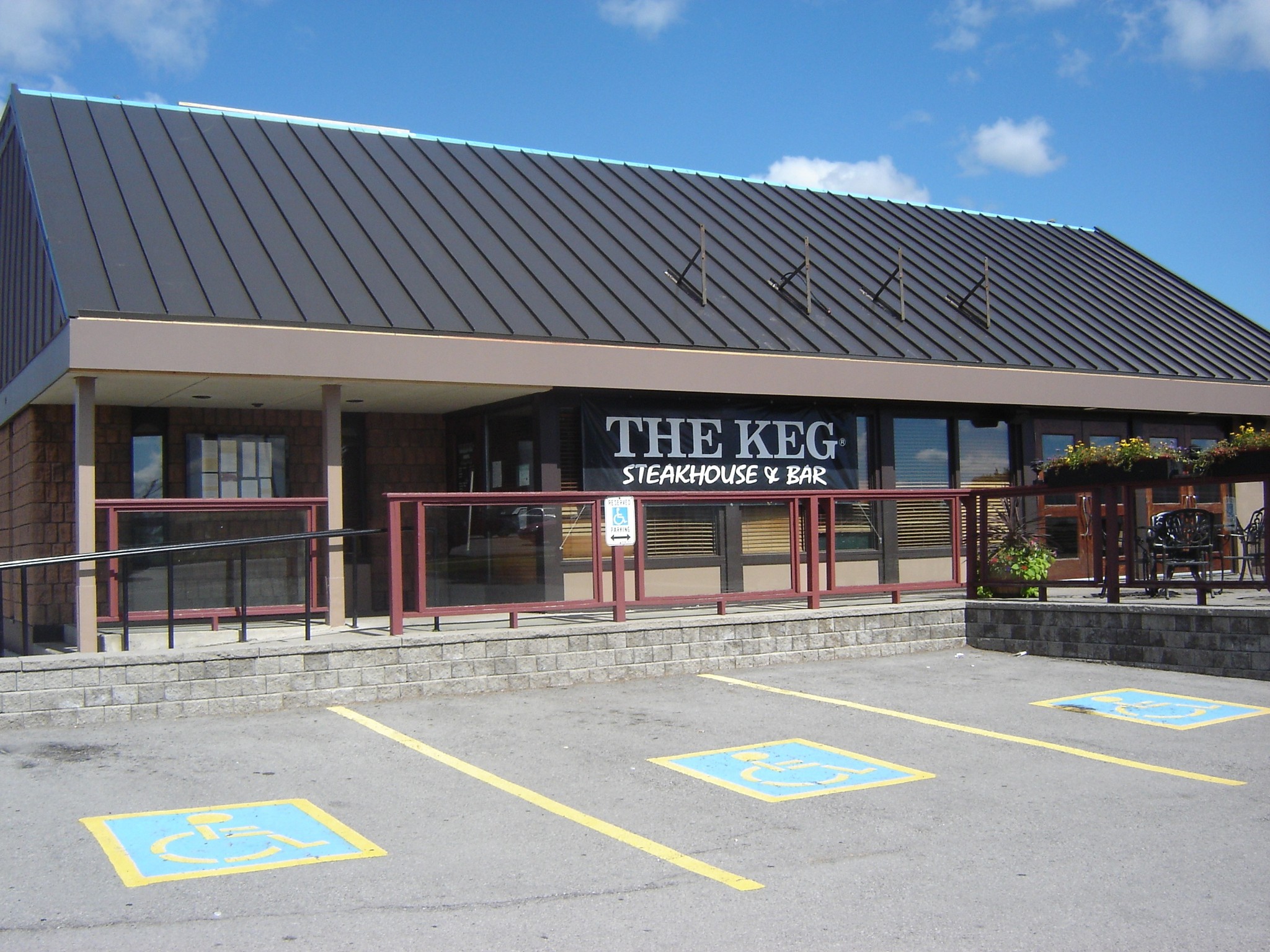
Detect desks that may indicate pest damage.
[1136,520,1236,598]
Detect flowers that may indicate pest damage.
[975,538,1058,599]
[1031,421,1270,478]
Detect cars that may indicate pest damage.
[458,505,557,540]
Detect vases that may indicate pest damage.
[1197,446,1270,484]
[979,560,1032,598]
[1114,457,1176,481]
[1042,461,1114,488]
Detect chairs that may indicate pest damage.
[1077,491,1270,601]
